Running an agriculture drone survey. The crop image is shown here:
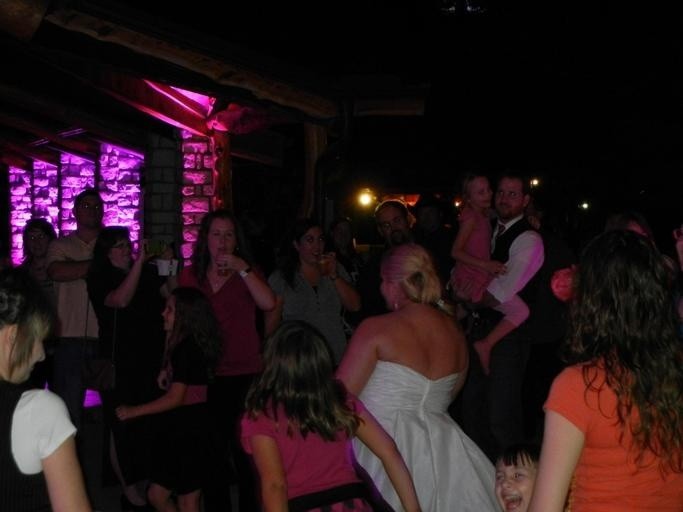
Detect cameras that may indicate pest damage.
[145,237,161,254]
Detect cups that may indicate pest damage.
[216,251,228,277]
[319,255,336,280]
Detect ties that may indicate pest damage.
[495,224,505,240]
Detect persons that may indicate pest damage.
[0,168,683,512]
